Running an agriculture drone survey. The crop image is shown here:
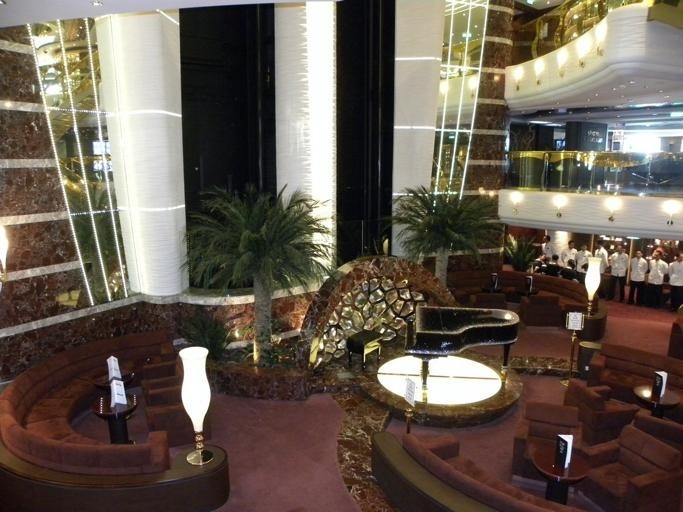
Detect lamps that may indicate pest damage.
[179,347,213,466]
[584,257,602,318]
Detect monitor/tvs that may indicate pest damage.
[555,140,566,151]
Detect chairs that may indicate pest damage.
[520,295,562,326]
[141,357,185,394]
[473,293,506,309]
[668,317,683,360]
[514,384,683,512]
[145,386,212,448]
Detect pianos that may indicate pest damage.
[405,307,520,402]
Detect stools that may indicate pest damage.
[346,329,384,369]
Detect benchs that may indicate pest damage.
[369,434,584,512]
[446,271,606,341]
[0,331,231,512]
[587,343,683,420]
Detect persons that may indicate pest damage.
[504,231,683,313]
